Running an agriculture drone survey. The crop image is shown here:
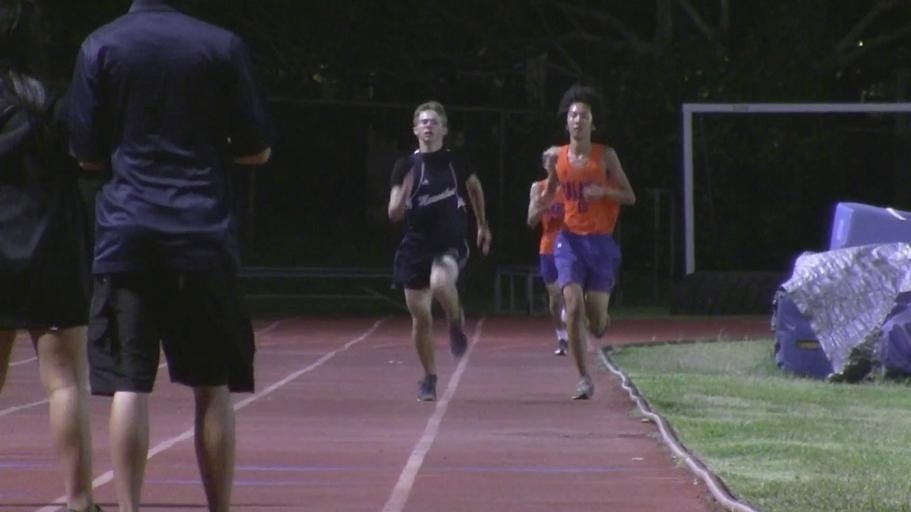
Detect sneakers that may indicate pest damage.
[417,377,438,403]
[572,381,595,401]
[448,308,469,356]
[555,341,570,358]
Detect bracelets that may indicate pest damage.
[602,186,612,200]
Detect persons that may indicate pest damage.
[2,2,107,511]
[537,85,640,401]
[385,99,494,401]
[70,2,282,511]
[525,145,581,358]
